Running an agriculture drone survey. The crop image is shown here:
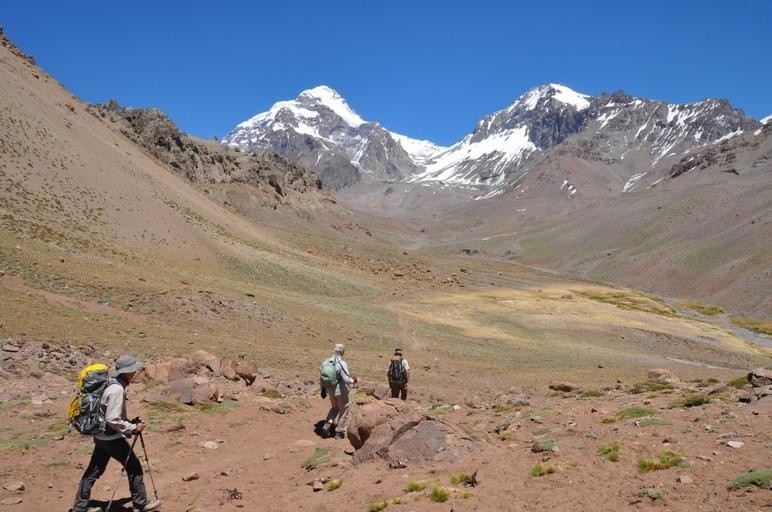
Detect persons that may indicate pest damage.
[319,342,358,439]
[71,353,162,511]
[386,347,410,401]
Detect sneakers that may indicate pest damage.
[322,422,347,439]
[132,499,161,512]
[78,506,101,512]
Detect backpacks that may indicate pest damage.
[389,357,406,384]
[68,363,108,436]
[320,362,338,387]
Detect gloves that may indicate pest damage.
[320,387,326,399]
[353,377,358,383]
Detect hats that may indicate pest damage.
[394,348,403,355]
[335,344,343,351]
[110,355,144,378]
